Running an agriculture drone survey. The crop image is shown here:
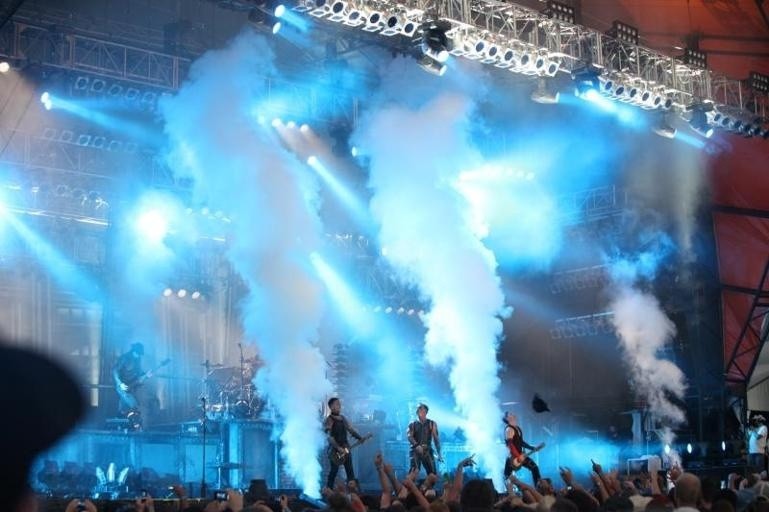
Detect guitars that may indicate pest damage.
[328,432,372,466]
[510,443,545,470]
[122,358,170,392]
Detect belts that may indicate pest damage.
[409,444,431,448]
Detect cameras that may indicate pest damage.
[214,491,228,501]
[77,500,85,508]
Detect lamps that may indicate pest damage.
[409,28,449,76]
[528,75,561,104]
[245,2,286,34]
[652,105,717,141]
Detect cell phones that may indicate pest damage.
[141,491,148,500]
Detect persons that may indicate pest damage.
[36,450,498,512]
[502,410,541,485]
[324,397,364,489]
[743,415,768,471]
[494,450,769,511]
[405,404,443,481]
[109,342,154,430]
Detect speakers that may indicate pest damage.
[627,458,648,475]
